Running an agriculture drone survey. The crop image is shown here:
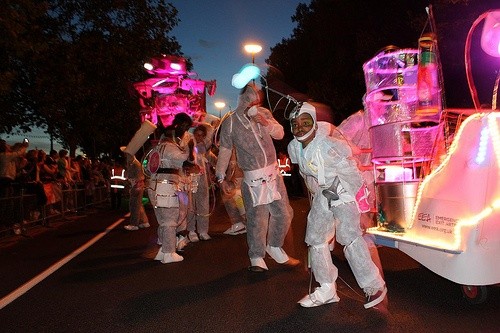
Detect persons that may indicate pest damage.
[120,147,150,230]
[287,101,389,310]
[214,84,295,274]
[210,121,249,237]
[0,137,125,238]
[182,124,209,241]
[145,126,189,262]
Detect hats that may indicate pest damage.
[289,102,316,125]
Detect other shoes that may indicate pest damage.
[138,222,150,228]
[155,249,183,264]
[189,232,199,242]
[157,237,178,245]
[250,257,268,270]
[124,225,138,230]
[299,286,340,307]
[200,232,211,240]
[363,283,388,309]
[177,235,190,250]
[266,245,289,264]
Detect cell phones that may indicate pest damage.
[24,138,29,142]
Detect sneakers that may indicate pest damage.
[223,222,247,235]
